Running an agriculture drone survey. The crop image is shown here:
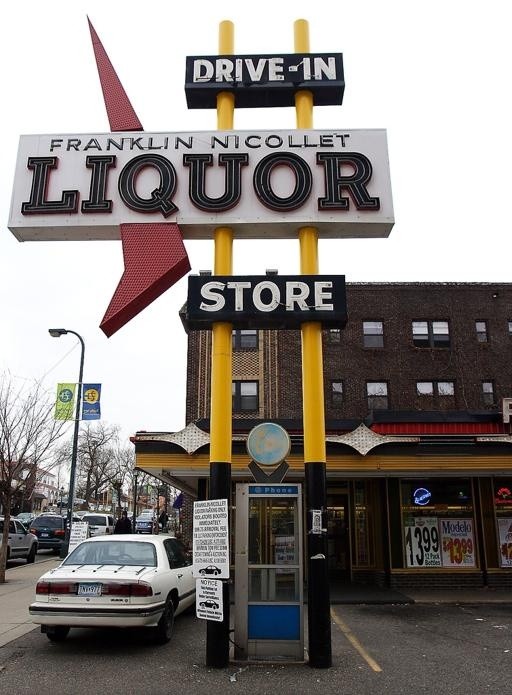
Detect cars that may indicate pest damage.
[0,495,162,572]
[199,565,222,576]
[198,600,220,610]
[25,525,194,645]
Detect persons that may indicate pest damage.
[113,511,132,534]
[158,510,168,530]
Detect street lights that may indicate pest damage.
[47,327,86,560]
